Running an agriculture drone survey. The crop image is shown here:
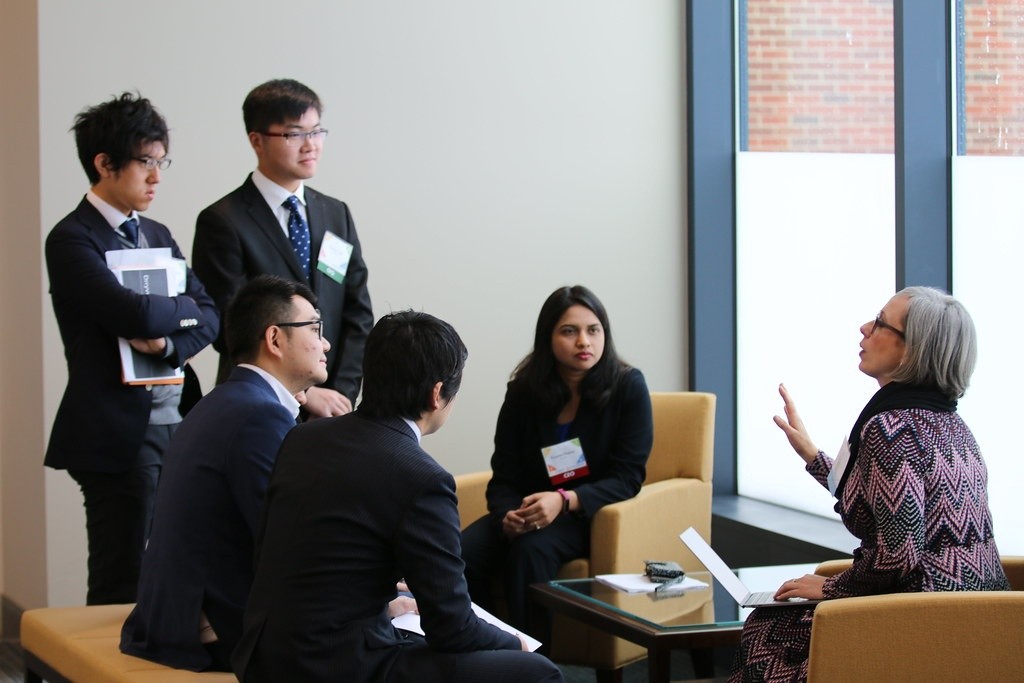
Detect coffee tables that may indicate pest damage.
[527,563,817,683]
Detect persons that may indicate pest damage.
[42,93,220,606]
[461,285,654,655]
[725,286,1012,683]
[233,311,564,683]
[118,273,331,676]
[191,78,374,425]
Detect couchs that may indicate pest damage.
[808,555,1024,683]
[455,391,716,683]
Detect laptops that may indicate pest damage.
[679,527,823,607]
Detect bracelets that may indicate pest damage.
[557,488,570,513]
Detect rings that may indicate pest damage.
[534,522,540,529]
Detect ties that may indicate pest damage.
[281,196,310,283]
[119,219,138,246]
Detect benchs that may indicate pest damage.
[22,604,238,683]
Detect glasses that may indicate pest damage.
[870,316,906,341]
[260,128,328,146]
[127,155,172,171]
[261,320,323,340]
